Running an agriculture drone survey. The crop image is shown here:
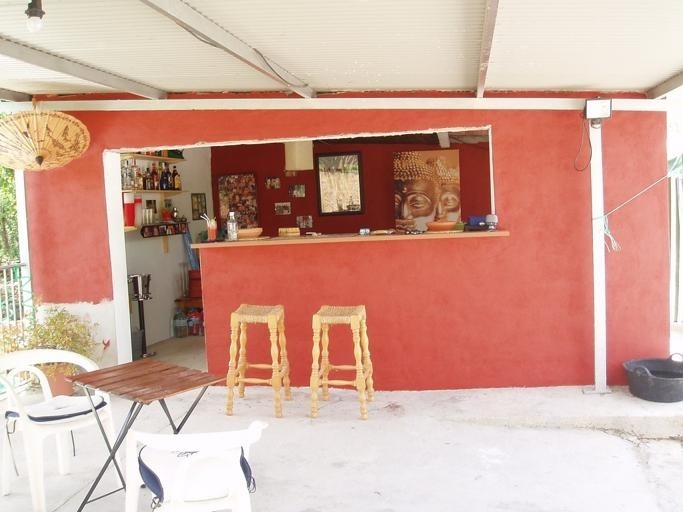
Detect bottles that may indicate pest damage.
[172,207,178,219]
[134,196,143,228]
[172,306,204,338]
[121,158,182,191]
[123,192,135,227]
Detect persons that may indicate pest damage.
[393,150,461,232]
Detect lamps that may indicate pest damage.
[573,96,612,172]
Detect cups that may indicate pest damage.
[141,208,155,226]
[206,220,217,242]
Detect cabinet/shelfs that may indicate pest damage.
[120,153,188,232]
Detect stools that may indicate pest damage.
[225,304,375,421]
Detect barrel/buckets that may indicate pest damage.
[188,269,202,299]
[129,273,151,300]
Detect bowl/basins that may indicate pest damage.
[621,351,683,403]
[236,227,263,239]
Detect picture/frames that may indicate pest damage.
[313,150,366,216]
